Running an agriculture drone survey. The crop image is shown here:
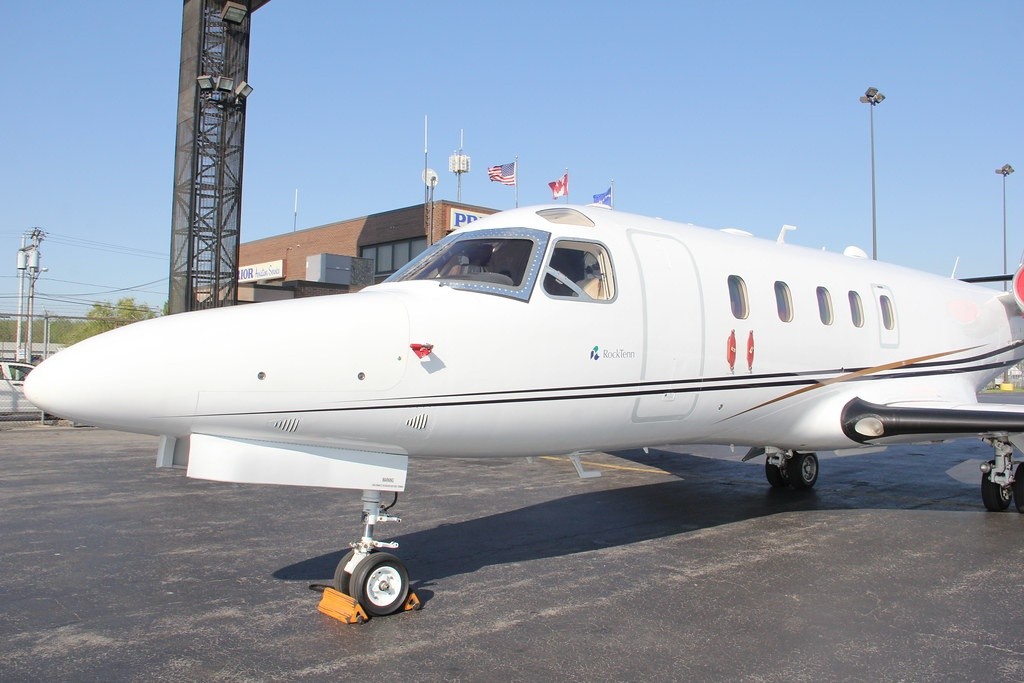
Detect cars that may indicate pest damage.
[0,359,59,420]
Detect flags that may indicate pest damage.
[488,162,517,186]
[549,174,570,199]
[592,186,612,204]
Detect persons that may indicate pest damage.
[435,253,486,275]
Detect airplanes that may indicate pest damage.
[21,199,1024,612]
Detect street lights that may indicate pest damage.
[994,163,1015,290]
[860,87,885,261]
[26,269,48,364]
